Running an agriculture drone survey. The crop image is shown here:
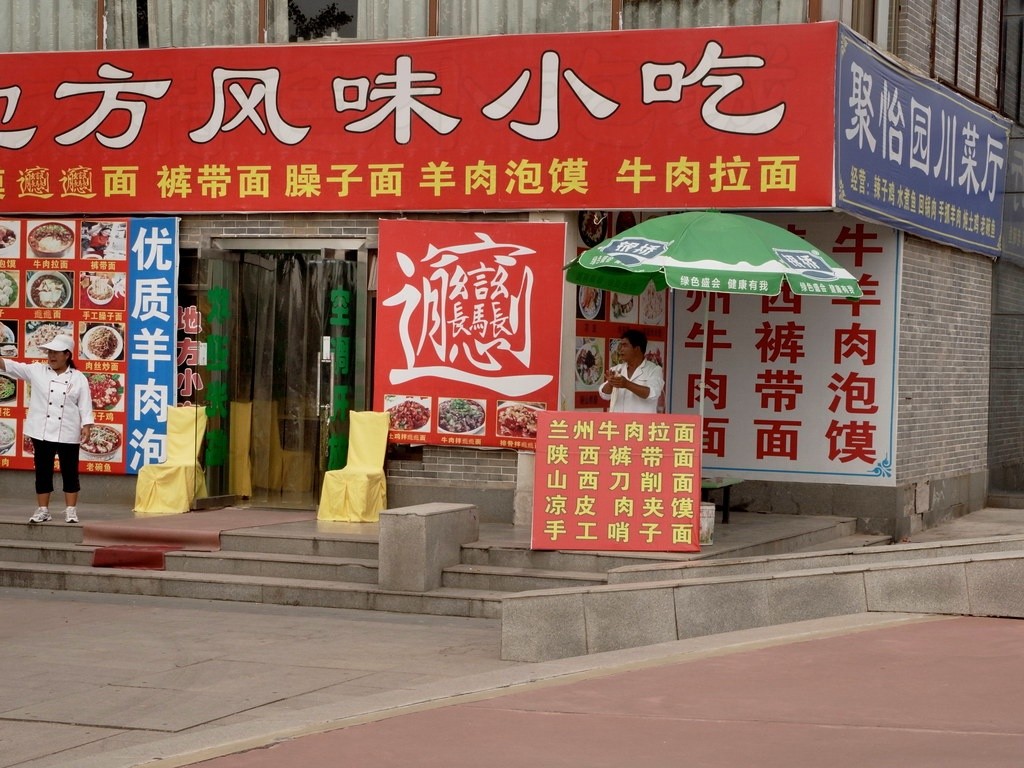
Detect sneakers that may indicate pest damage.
[63,507,79,523]
[29,507,52,523]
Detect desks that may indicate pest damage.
[702,477,744,525]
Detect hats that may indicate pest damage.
[38,334,75,354]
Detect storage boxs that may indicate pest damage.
[698,502,716,545]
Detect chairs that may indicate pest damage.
[229,403,252,501]
[133,404,209,514]
[317,410,391,523]
[252,401,315,492]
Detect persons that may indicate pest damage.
[0,334,95,522]
[599,328,666,414]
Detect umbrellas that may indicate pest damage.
[557,208,863,474]
